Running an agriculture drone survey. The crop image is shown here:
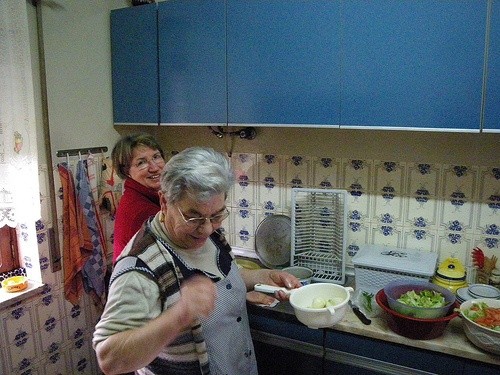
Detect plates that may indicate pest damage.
[455,284,500,305]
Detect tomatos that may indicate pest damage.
[471,307,500,330]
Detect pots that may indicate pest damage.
[432,258,466,293]
[254,283,354,329]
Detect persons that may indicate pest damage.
[110,132,274,305]
[92,147,303,375]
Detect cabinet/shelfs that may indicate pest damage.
[109,0,500,133]
[249,314,500,375]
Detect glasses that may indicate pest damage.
[130,151,164,172]
[174,202,231,228]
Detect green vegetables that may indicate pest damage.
[362,291,375,312]
[463,302,489,321]
[392,289,446,319]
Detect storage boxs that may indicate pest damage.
[352,244,438,293]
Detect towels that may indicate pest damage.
[56,155,117,309]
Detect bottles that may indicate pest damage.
[490,268,500,289]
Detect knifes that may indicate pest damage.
[348,299,373,324]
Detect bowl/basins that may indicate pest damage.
[376,284,462,339]
[281,266,314,286]
[384,279,456,318]
[1,276,29,292]
[459,298,500,355]
